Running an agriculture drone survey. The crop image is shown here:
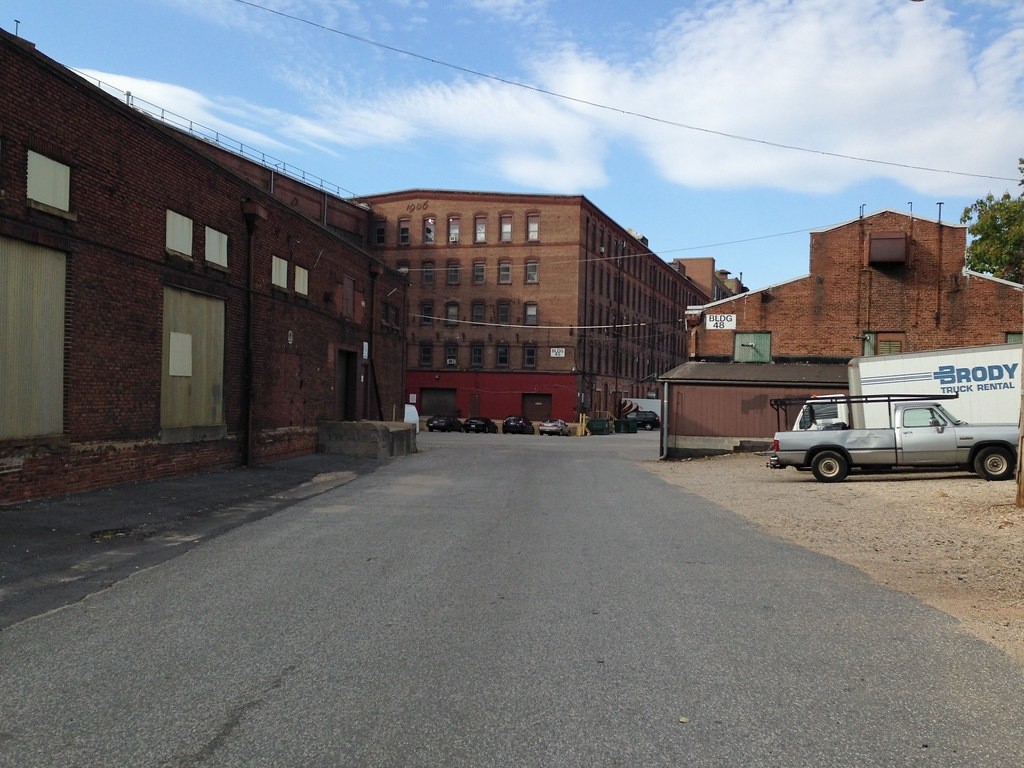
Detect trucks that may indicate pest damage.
[620,398,660,423]
[789,342,1024,433]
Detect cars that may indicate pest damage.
[463,416,498,433]
[502,416,535,435]
[425,415,464,432]
[538,418,571,437]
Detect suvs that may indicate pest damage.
[626,410,661,430]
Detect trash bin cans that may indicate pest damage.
[588,419,610,435]
[615,418,638,433]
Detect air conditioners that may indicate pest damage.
[449,235,458,242]
[601,247,605,252]
[447,358,456,364]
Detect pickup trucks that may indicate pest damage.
[762,400,1020,482]
